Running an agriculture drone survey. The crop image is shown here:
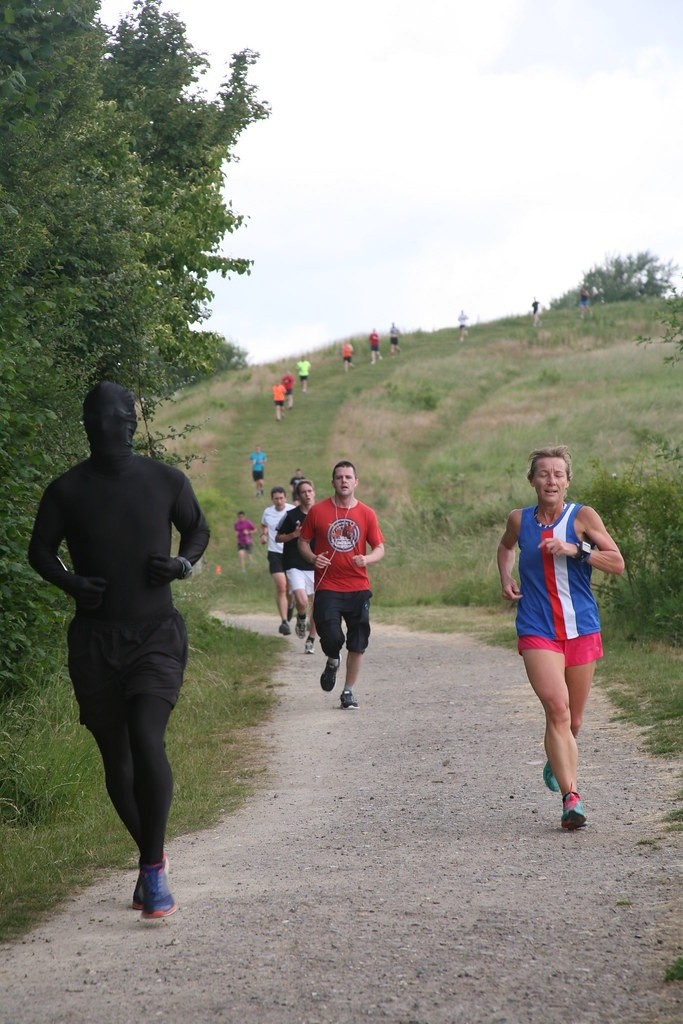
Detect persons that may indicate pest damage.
[300,460,387,708]
[369,328,384,365]
[274,480,316,653]
[531,295,542,328]
[260,486,296,635]
[498,447,625,827]
[234,511,257,575]
[290,468,305,506]
[579,286,594,320]
[458,310,469,341]
[272,379,288,422]
[248,444,267,496]
[283,370,294,409]
[389,321,402,357]
[26,381,209,921]
[340,338,355,373]
[295,356,312,392]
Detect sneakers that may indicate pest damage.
[295,614,307,639]
[560,781,586,828]
[320,654,342,691]
[305,640,314,654]
[131,858,168,909]
[139,852,177,917]
[340,691,359,709]
[542,761,559,792]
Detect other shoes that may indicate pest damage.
[279,624,290,636]
[287,602,294,621]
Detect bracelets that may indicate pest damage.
[260,533,267,538]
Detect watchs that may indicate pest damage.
[174,556,192,579]
[575,540,592,562]
